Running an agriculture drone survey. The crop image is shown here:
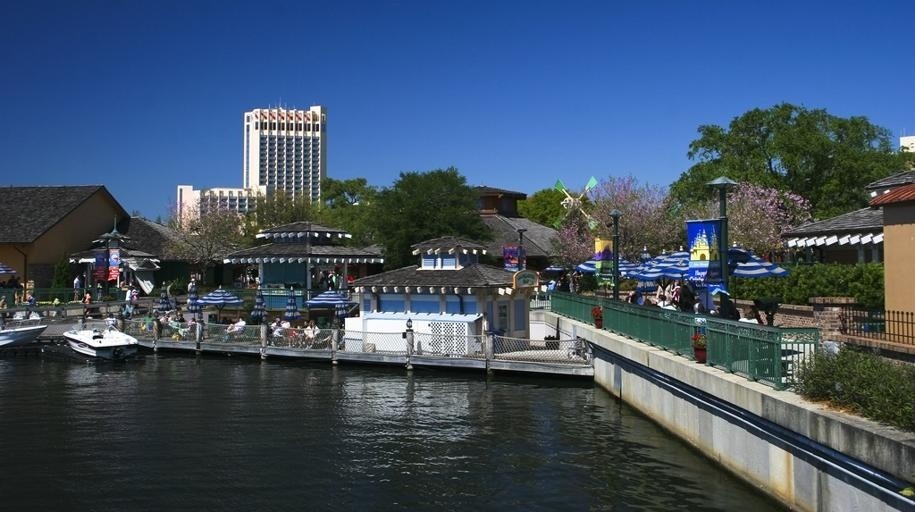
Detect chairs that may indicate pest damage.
[234,327,248,344]
[184,324,196,342]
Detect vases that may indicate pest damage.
[595,319,602,328]
[693,346,706,363]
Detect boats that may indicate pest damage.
[0,318,50,349]
[62,321,142,363]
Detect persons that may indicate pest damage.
[141,310,347,351]
[2,272,141,332]
[321,270,355,291]
[624,279,765,328]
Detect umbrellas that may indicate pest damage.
[724,239,791,306]
[0,262,18,275]
[574,251,637,296]
[544,263,571,272]
[622,248,676,307]
[634,245,660,301]
[636,245,690,287]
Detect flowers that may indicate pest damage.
[691,334,706,347]
[591,306,602,318]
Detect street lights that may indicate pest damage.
[610,207,623,300]
[515,229,525,249]
[706,176,743,318]
